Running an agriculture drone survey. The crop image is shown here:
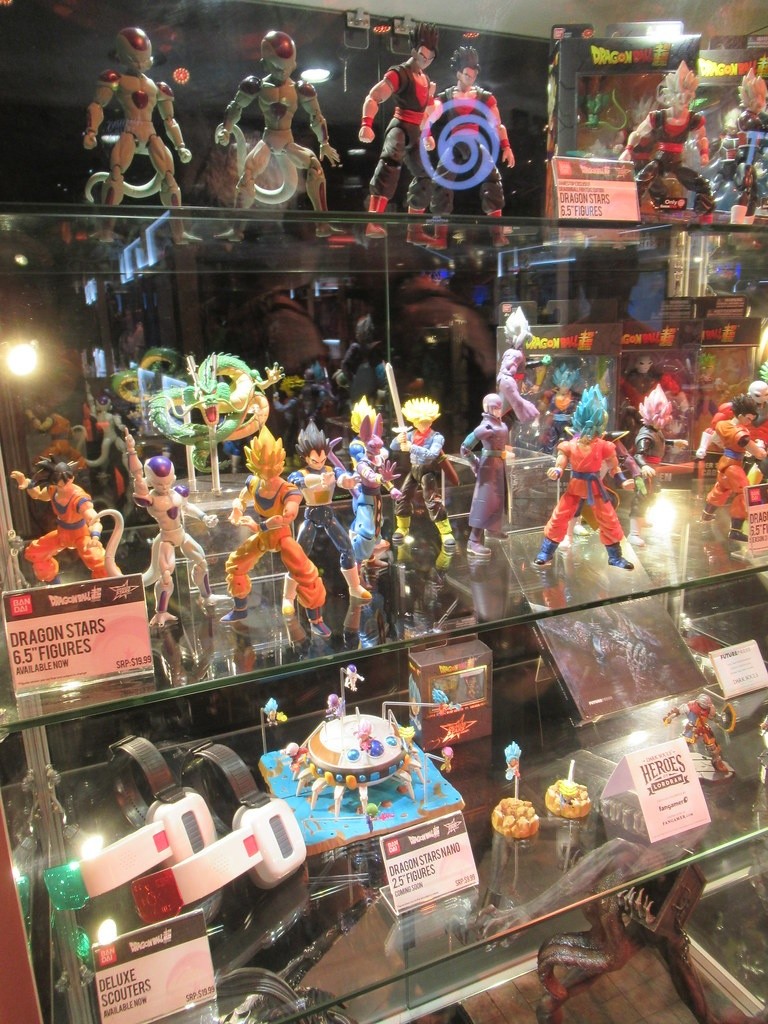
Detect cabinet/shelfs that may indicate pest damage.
[0,203,768,1024]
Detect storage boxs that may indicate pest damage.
[405,614,494,751]
[496,298,758,490]
[548,21,768,217]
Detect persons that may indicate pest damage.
[493,663,582,761]
[115,229,656,482]
